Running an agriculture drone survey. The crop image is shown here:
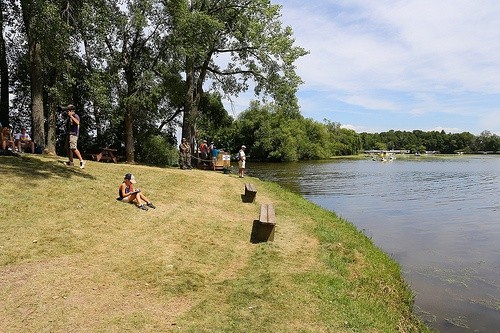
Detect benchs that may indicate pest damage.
[255,203,276,242]
[244,183,257,203]
[89,153,101,157]
[114,156,123,159]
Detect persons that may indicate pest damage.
[66,104,84,168]
[119,173,156,211]
[179,137,224,170]
[238,145,246,178]
[0,126,35,154]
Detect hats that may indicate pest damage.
[203,140,207,143]
[242,145,246,148]
[124,174,137,183]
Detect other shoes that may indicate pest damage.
[80,160,84,169]
[64,161,74,166]
[147,202,156,209]
[136,204,148,211]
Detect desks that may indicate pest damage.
[99,147,118,164]
